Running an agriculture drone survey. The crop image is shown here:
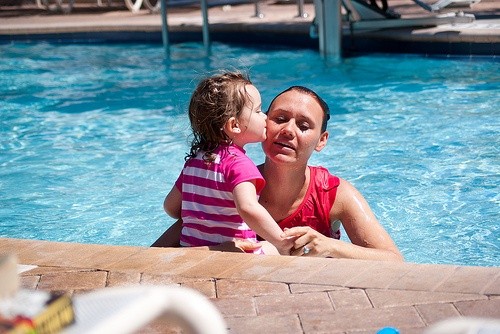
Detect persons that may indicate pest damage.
[163,71,300,255]
[152,86,402,261]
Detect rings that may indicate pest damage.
[303,246,309,254]
